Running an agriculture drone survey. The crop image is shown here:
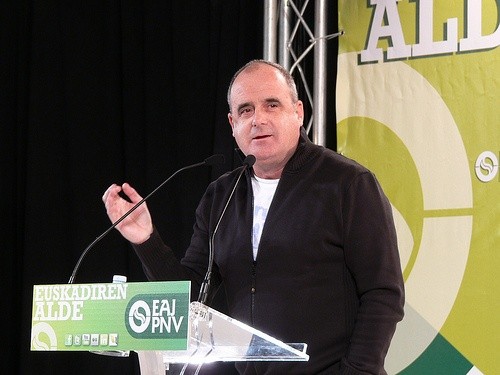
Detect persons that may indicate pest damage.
[103,60,405,375]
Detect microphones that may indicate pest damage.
[197,154,257,306]
[67,154,224,283]
